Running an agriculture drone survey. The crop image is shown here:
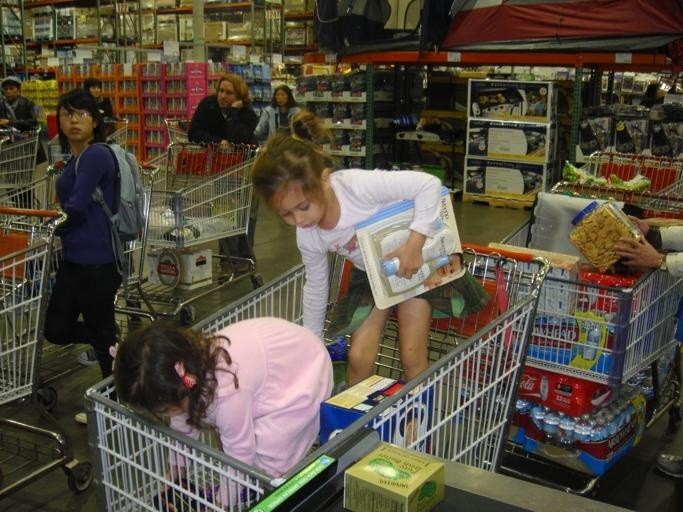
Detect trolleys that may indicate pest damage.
[0,117,194,337]
[469,151,683,501]
[82,237,552,512]
[0,203,94,498]
[113,138,266,328]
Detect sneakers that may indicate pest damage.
[656,454,683,478]
[79,349,99,366]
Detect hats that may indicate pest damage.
[1,76,21,87]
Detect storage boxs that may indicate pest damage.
[316,373,433,454]
[340,438,446,512]
[143,245,212,291]
[480,241,578,319]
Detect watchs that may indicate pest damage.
[658,253,668,274]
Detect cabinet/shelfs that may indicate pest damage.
[0,0,683,215]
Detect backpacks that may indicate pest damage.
[75,142,146,241]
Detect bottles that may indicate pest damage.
[506,310,617,375]
[566,198,642,274]
[453,346,674,477]
[578,118,681,160]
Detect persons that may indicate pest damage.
[249,128,446,398]
[109,310,335,512]
[41,89,126,426]
[638,84,664,109]
[253,84,303,148]
[607,210,682,478]
[82,77,118,146]
[187,71,262,286]
[1,73,47,216]
